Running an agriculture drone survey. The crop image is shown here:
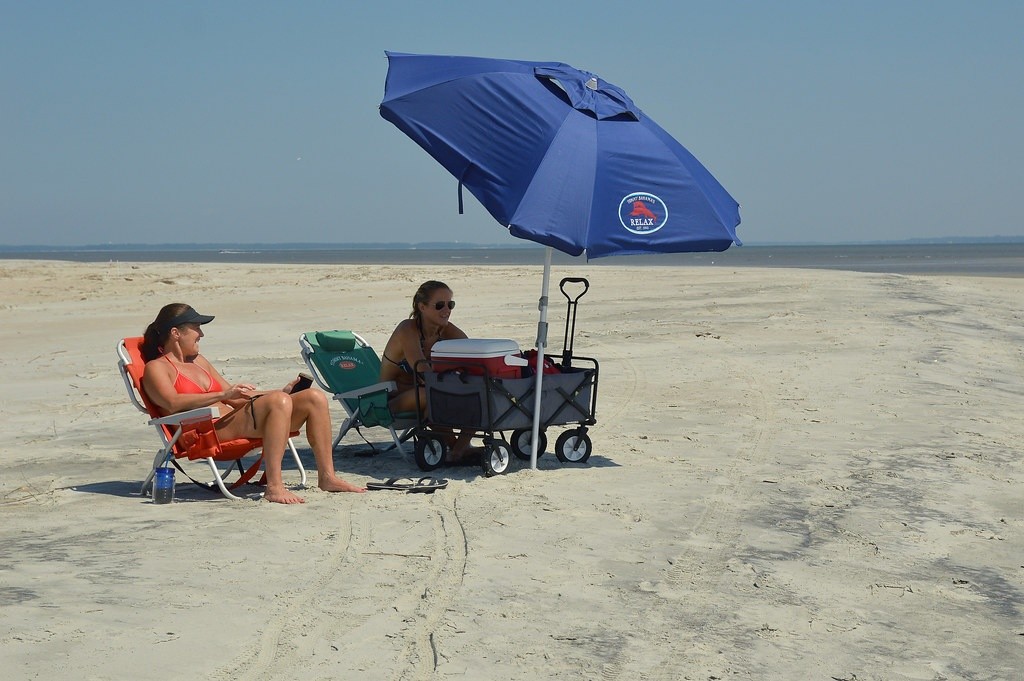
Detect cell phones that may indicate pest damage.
[289,373,314,394]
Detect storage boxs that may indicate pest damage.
[431,340,522,380]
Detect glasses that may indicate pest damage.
[427,300,455,310]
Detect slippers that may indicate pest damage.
[408,477,448,492]
[366,477,424,490]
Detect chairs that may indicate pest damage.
[117,334,308,500]
[297,331,427,467]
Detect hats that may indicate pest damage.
[157,308,215,336]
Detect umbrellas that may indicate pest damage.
[375,51,742,469]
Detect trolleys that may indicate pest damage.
[413,276,600,477]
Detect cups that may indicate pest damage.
[153,467,177,504]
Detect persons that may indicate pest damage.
[141,303,365,504]
[380,280,478,466]
